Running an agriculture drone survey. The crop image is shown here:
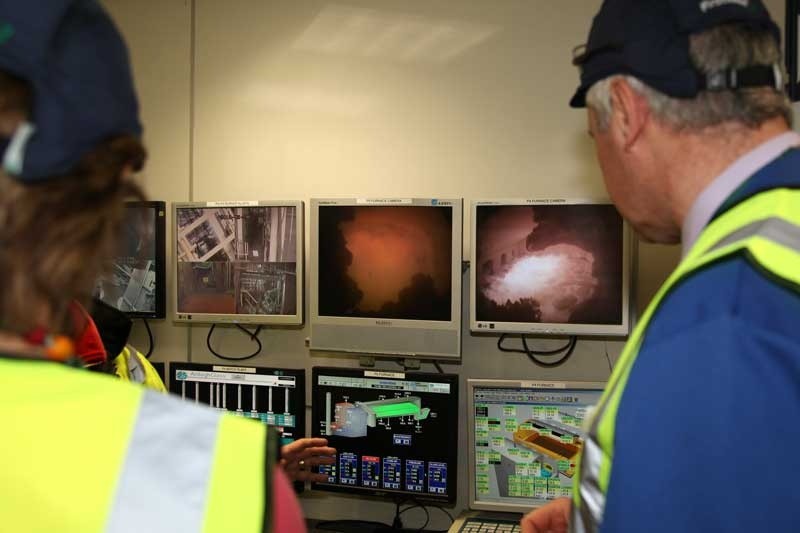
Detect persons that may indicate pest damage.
[86,296,336,481]
[0,0,305,533]
[522,0,800,533]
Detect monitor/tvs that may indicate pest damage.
[469,198,631,339]
[170,200,305,326]
[95,201,166,319]
[465,378,607,514]
[311,366,458,510]
[309,197,463,363]
[167,361,305,493]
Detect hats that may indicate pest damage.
[569,0,784,108]
[0,0,137,179]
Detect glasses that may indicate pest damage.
[570,43,622,74]
